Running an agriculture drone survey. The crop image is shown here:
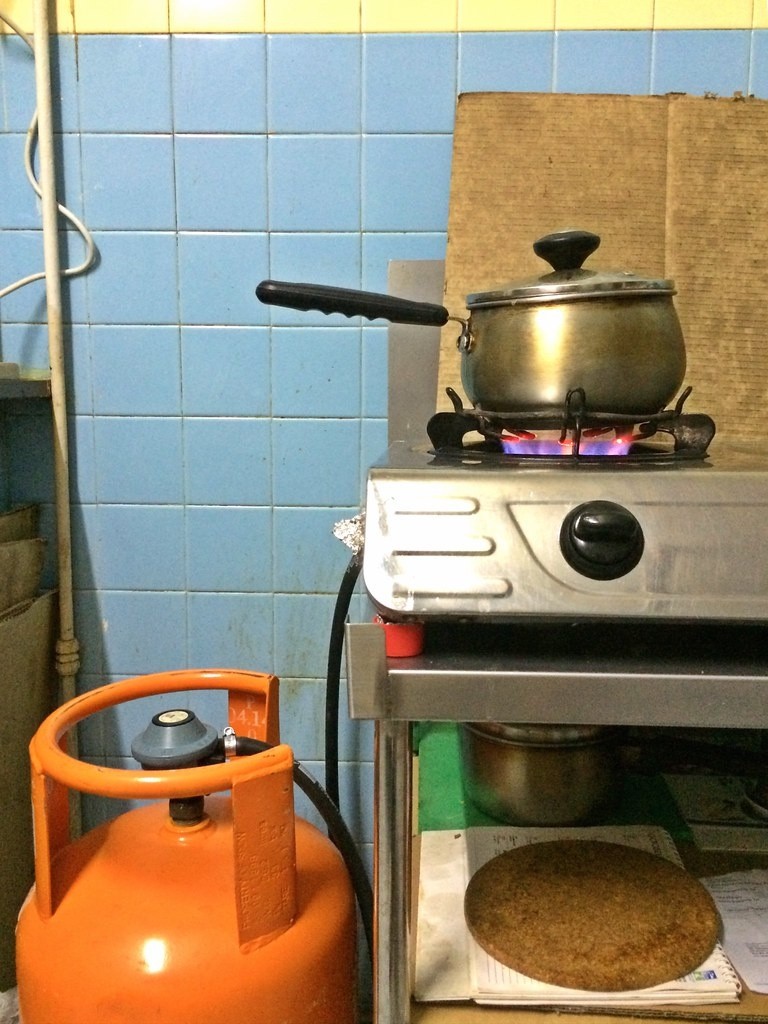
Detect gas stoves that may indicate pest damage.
[361,389,768,628]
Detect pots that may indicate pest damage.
[460,719,620,829]
[256,228,687,415]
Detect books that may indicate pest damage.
[465,824,744,1006]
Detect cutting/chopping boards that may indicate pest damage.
[463,839,721,993]
